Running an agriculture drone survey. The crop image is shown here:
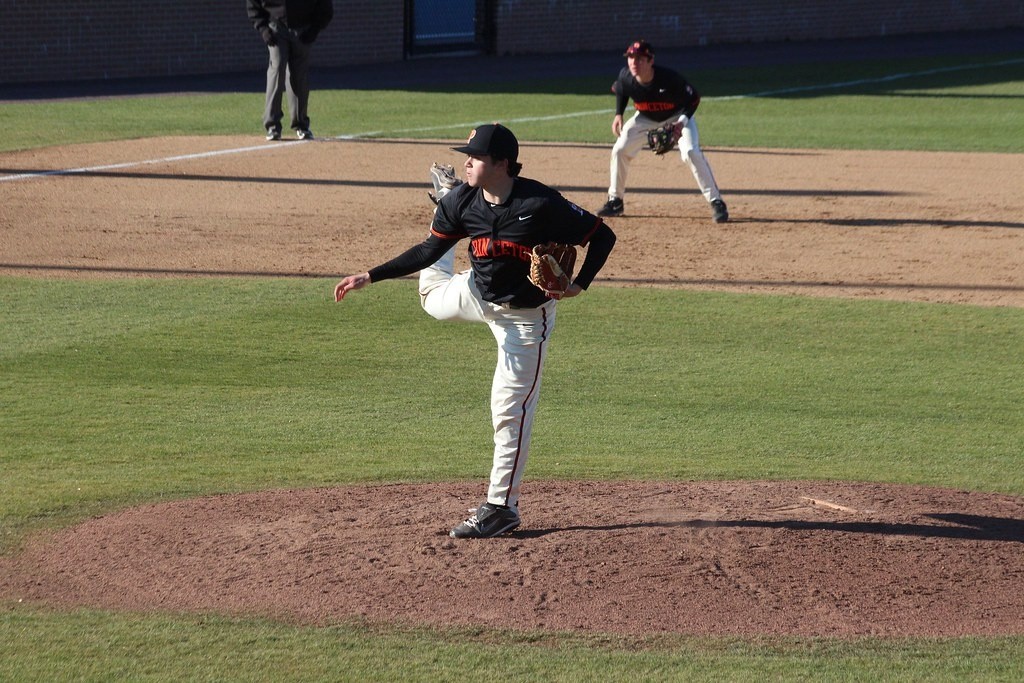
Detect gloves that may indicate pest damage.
[298,24,322,45]
[262,27,274,46]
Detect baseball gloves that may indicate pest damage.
[523,241,577,301]
[647,122,682,155]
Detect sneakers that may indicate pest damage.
[296,130,314,140]
[266,126,281,140]
[449,501,521,538]
[597,196,624,216]
[429,162,464,204]
[712,200,728,224]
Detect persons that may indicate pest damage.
[247,0,334,141]
[333,122,616,540]
[593,39,728,223]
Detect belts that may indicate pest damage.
[493,301,519,310]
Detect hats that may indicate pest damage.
[452,121,520,161]
[622,39,654,57]
